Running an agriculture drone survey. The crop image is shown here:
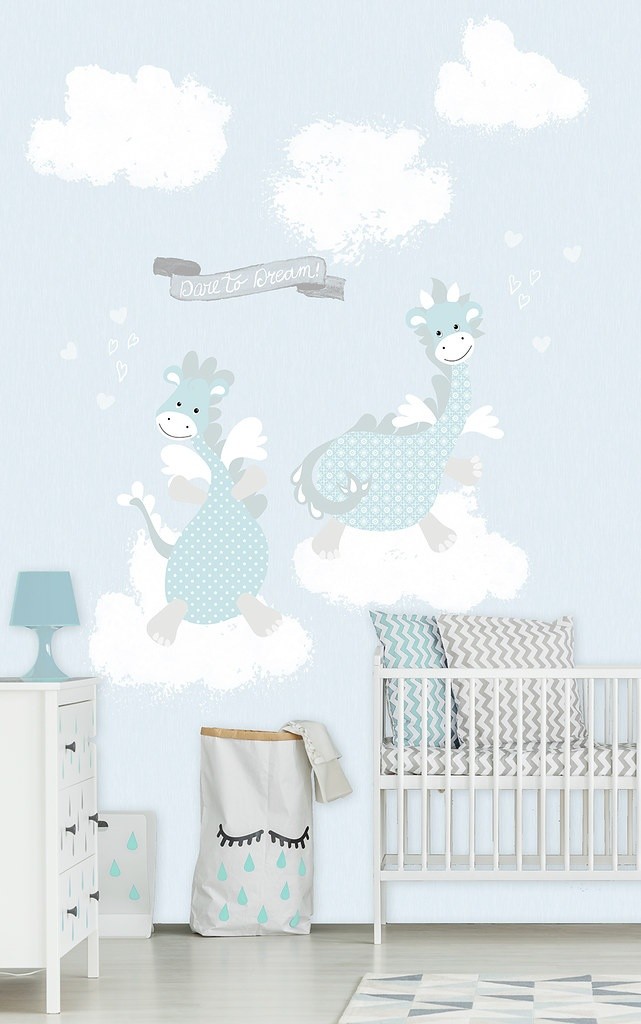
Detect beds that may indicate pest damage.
[374,645,640,945]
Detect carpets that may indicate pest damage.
[336,968,641,1024]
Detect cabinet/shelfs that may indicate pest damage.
[0,678,101,1016]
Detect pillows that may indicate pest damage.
[430,611,594,750]
[368,610,461,748]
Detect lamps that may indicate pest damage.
[8,571,80,681]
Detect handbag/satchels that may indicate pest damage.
[189,727,317,935]
[95,813,155,939]
